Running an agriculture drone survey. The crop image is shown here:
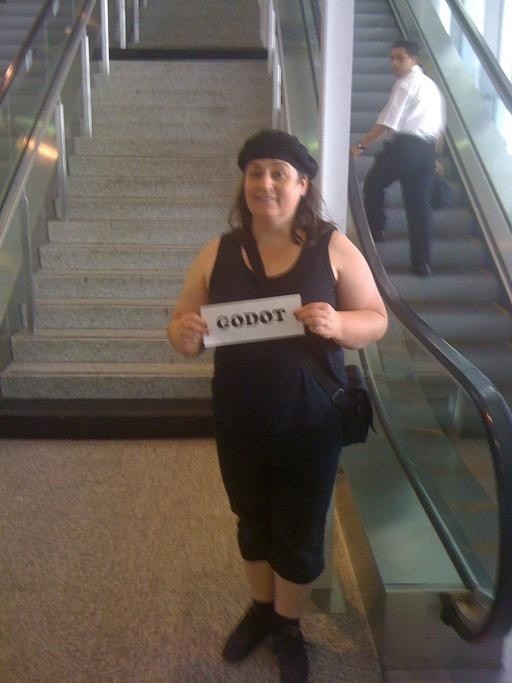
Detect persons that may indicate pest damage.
[163,127,388,682]
[350,38,450,278]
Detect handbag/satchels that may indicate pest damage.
[341,365,373,443]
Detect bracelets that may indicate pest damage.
[355,140,365,151]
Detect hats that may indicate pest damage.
[238,129,318,179]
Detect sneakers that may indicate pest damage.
[274,620,308,682]
[223,604,273,660]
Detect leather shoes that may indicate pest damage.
[370,227,384,241]
[410,264,430,276]
